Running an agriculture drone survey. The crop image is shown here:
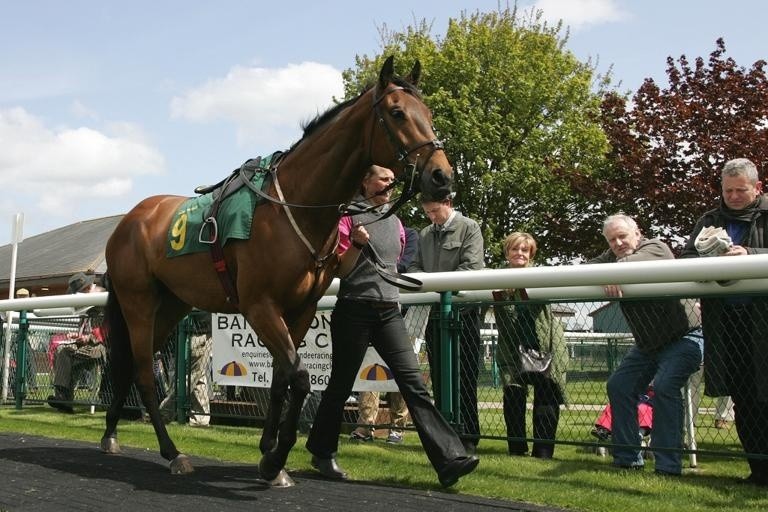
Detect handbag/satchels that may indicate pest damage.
[510,345,554,384]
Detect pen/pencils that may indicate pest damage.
[69,330,71,341]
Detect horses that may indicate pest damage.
[100,53,456,490]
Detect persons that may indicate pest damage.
[403,188,487,456]
[46,271,114,413]
[679,157,767,489]
[493,231,570,461]
[347,226,419,447]
[584,212,705,480]
[143,306,213,427]
[305,166,481,488]
[591,372,657,440]
[682,362,733,429]
[3,286,40,392]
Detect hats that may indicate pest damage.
[66,273,95,294]
[17,289,28,294]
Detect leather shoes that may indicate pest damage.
[438,456,480,487]
[312,454,348,479]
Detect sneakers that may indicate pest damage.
[351,429,405,445]
[48,395,74,408]
[591,428,607,440]
